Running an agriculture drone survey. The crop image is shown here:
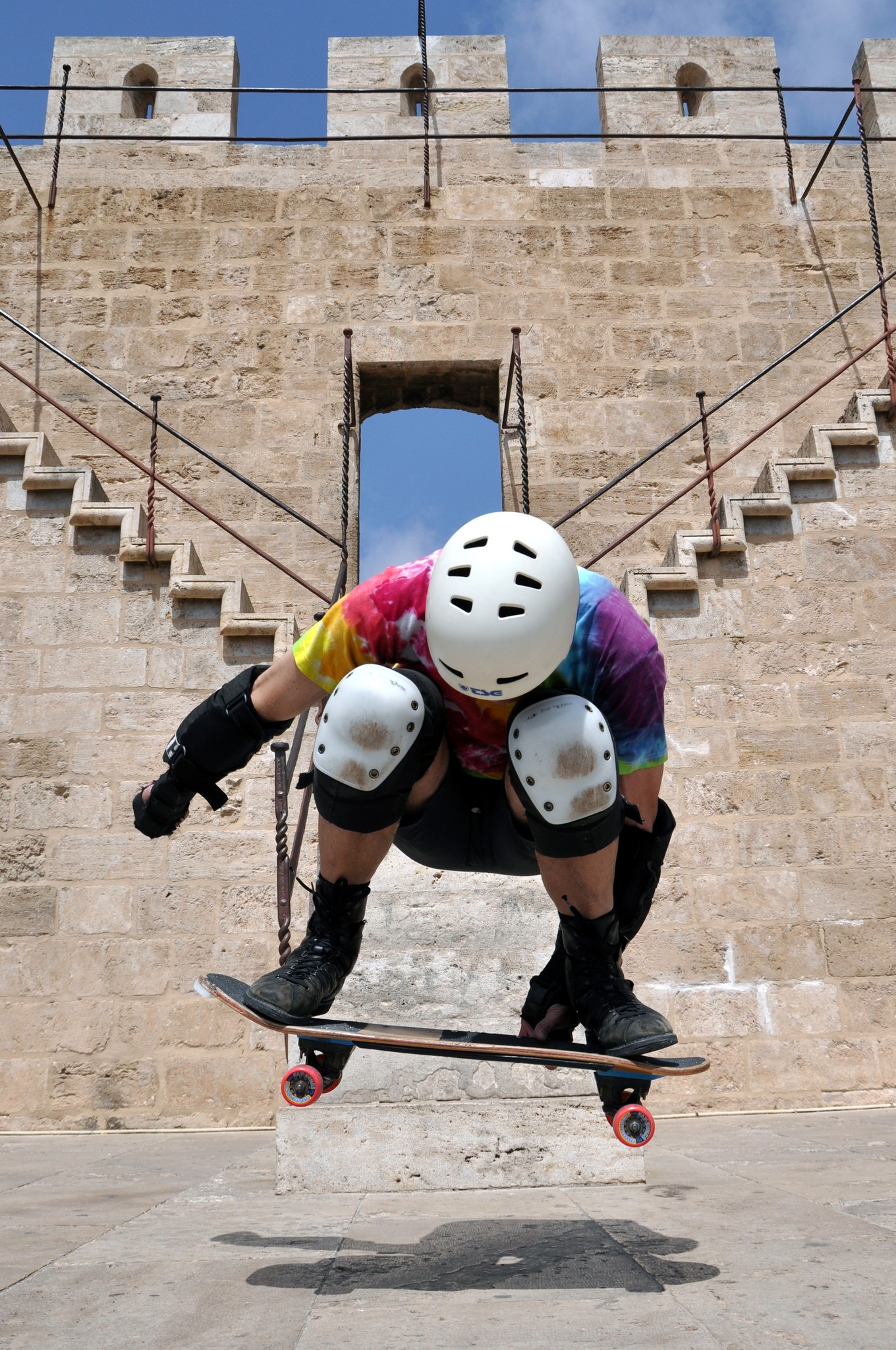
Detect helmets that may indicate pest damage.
[426,513,580,701]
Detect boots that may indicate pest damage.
[244,870,372,1023]
[555,894,677,1055]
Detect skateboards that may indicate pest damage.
[199,964,715,1150]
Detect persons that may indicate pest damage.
[129,510,680,1057]
